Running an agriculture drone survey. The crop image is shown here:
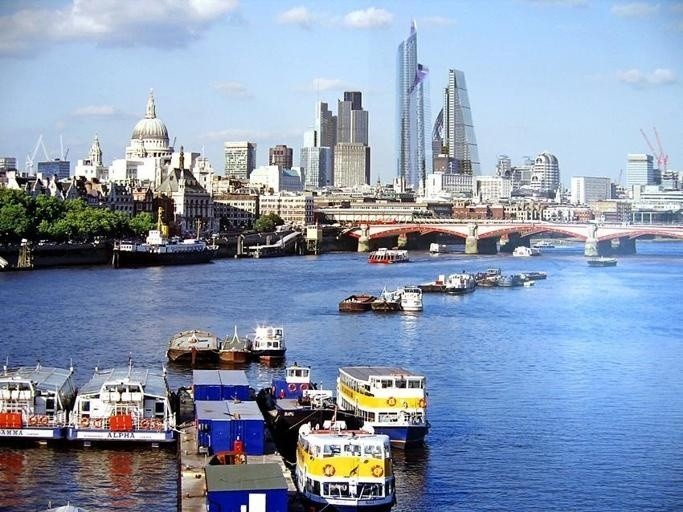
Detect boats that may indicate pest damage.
[165,330,221,363]
[586,255,616,267]
[335,364,431,452]
[428,243,447,253]
[0,439,62,510]
[296,424,396,512]
[0,357,77,444]
[256,363,353,466]
[512,246,539,257]
[367,246,409,264]
[337,266,548,313]
[534,241,555,249]
[251,325,286,367]
[67,448,171,508]
[427,252,447,258]
[219,359,249,372]
[68,358,175,449]
[388,443,427,509]
[111,230,218,270]
[217,324,250,364]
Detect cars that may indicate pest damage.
[18,237,79,247]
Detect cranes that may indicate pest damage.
[638,124,668,172]
[24,133,42,178]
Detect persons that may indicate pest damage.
[177,387,190,399]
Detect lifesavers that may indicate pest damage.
[29,416,38,424]
[140,419,150,428]
[300,383,308,391]
[93,419,103,428]
[80,417,90,427]
[289,383,297,393]
[323,464,335,476]
[40,416,48,425]
[154,421,162,431]
[371,465,383,477]
[388,396,397,406]
[420,399,428,408]
